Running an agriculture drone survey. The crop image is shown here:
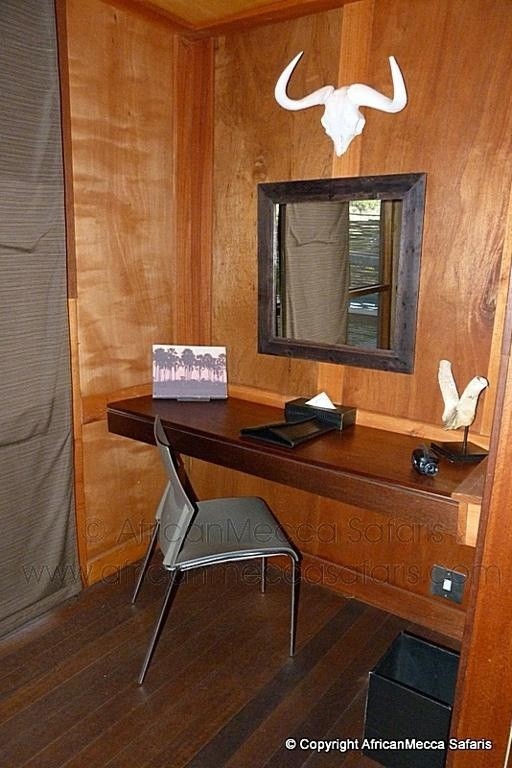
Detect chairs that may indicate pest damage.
[129,417,298,682]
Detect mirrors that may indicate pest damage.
[258,172,428,375]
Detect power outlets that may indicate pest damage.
[429,563,466,604]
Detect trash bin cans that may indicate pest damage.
[363,630,462,766]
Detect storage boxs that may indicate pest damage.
[364,632,464,766]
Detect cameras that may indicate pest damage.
[411,446,441,477]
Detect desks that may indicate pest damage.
[108,391,482,548]
